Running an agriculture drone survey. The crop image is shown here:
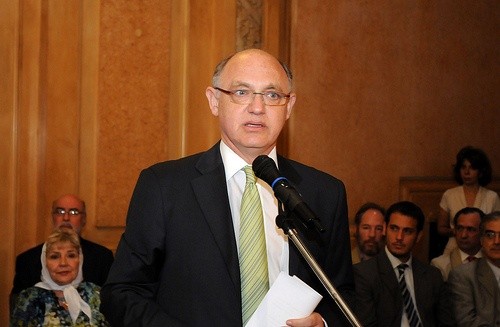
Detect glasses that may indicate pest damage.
[52,209,88,216]
[215,86,290,107]
[481,232,500,239]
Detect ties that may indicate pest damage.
[238,164,270,327]
[467,256,475,261]
[397,263,421,327]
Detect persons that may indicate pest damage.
[448,211,500,327]
[431,207,486,281]
[9,227,105,327]
[353,201,456,327]
[99,48,356,327]
[10,193,115,314]
[437,145,500,236]
[350,203,387,264]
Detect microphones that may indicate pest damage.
[252,154,324,233]
